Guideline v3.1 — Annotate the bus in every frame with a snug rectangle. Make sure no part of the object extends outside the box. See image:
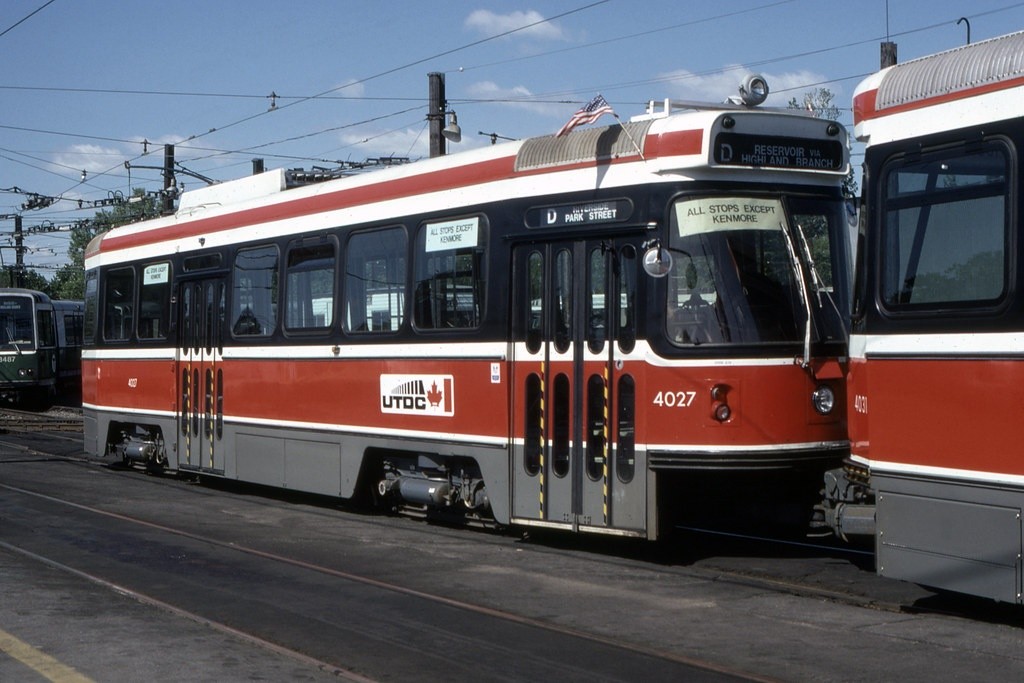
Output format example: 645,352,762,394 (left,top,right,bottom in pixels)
0,287,86,391
847,30,1024,607
83,74,900,545
